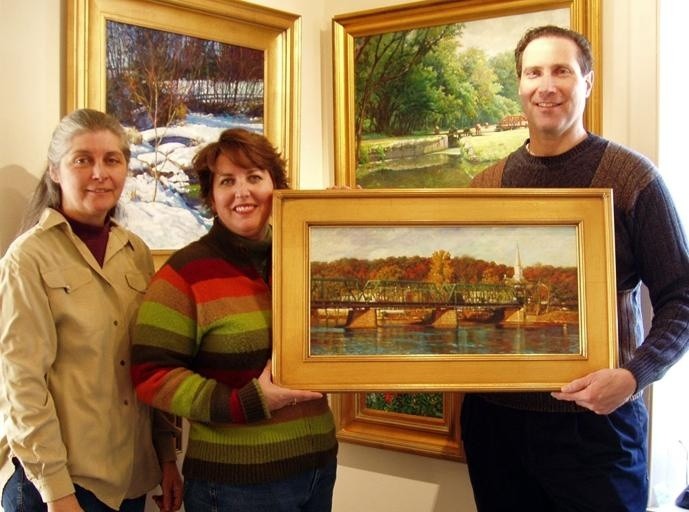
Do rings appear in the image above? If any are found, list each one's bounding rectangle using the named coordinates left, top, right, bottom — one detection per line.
left=292, top=398, right=298, bottom=405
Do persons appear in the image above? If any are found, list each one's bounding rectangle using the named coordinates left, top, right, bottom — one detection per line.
left=128, top=128, right=368, bottom=510
left=0, top=108, right=187, bottom=511
left=450, top=23, right=688, bottom=511
